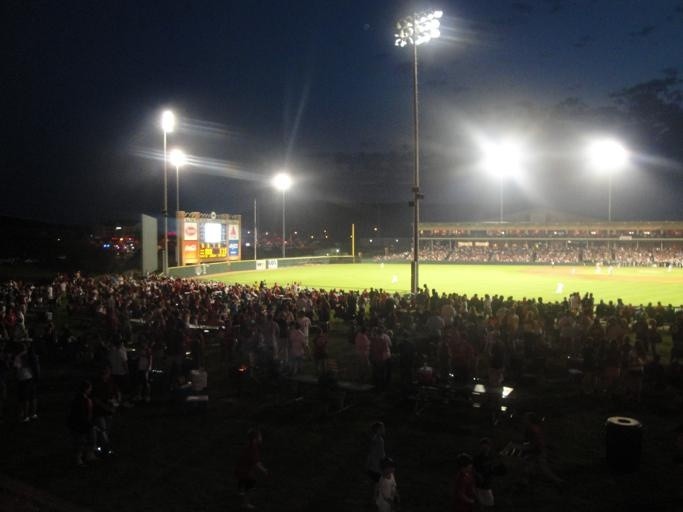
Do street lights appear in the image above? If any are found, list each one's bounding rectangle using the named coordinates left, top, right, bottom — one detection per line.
left=158, top=109, right=176, bottom=275
left=589, top=139, right=626, bottom=223
left=476, top=139, right=519, bottom=223
left=395, top=6, right=448, bottom=298
left=169, top=148, right=188, bottom=265
left=271, top=172, right=294, bottom=257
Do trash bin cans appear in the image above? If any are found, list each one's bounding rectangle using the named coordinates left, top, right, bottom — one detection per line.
left=52, top=254, right=67, bottom=272
left=607, top=416, right=642, bottom=476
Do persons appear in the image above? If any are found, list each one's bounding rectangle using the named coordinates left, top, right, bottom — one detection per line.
left=1, top=270, right=682, bottom=511
left=378, top=241, right=683, bottom=268
